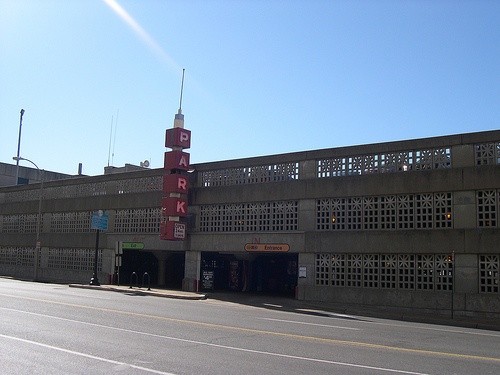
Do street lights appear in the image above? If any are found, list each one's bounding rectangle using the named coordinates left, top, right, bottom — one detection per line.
left=15, top=109, right=25, bottom=185
left=13, top=156, right=43, bottom=282
